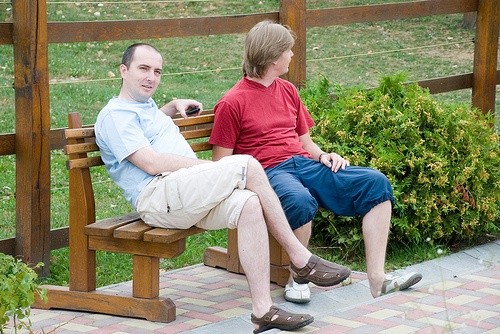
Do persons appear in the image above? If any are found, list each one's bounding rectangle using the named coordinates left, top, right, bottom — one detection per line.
left=94, top=44, right=350, bottom=334
left=208, top=18, right=423, bottom=299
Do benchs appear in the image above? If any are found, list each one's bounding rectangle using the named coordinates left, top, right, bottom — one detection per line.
left=30, top=108, right=292, bottom=323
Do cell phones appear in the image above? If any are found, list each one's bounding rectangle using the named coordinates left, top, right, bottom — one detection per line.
left=186, top=107, right=200, bottom=115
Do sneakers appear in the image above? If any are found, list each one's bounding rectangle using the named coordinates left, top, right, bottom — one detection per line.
left=251, top=305, right=315, bottom=334
left=378, top=272, right=422, bottom=296
left=285, top=281, right=311, bottom=303
left=290, top=254, right=350, bottom=287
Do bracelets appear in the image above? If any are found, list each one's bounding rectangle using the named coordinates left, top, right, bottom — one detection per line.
left=319, top=152, right=326, bottom=162
